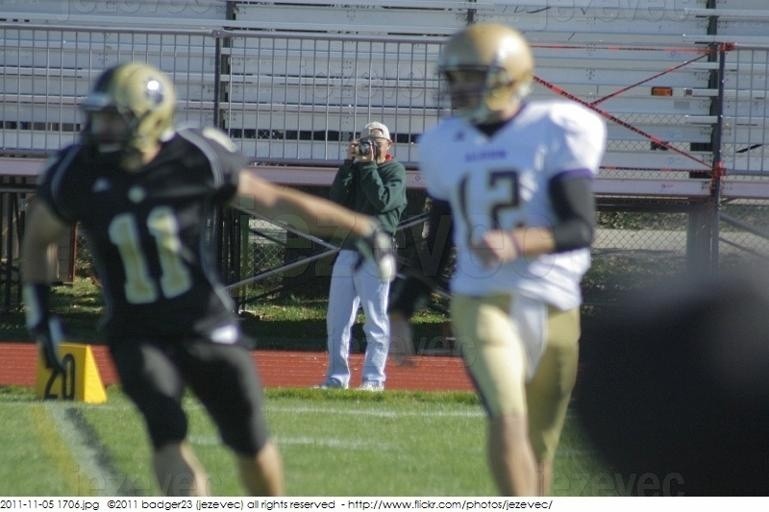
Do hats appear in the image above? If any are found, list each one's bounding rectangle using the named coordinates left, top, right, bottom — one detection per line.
left=360, top=122, right=391, bottom=143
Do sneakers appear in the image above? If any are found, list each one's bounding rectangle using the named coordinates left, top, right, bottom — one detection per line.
left=312, top=378, right=349, bottom=390
left=354, top=381, right=385, bottom=392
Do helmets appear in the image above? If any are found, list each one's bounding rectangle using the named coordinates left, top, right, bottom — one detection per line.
left=433, top=23, right=536, bottom=129
left=74, top=63, right=178, bottom=174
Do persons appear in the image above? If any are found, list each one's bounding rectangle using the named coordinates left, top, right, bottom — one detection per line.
left=19, top=58, right=399, bottom=497
left=311, top=119, right=409, bottom=392
left=387, top=23, right=609, bottom=497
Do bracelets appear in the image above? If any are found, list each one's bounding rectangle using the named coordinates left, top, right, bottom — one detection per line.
left=508, top=229, right=525, bottom=259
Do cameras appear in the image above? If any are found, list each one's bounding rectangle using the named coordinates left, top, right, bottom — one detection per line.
left=359, top=138, right=377, bottom=156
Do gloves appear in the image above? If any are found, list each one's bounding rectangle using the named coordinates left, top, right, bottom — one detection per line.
left=25, top=309, right=75, bottom=376
left=353, top=215, right=400, bottom=285
